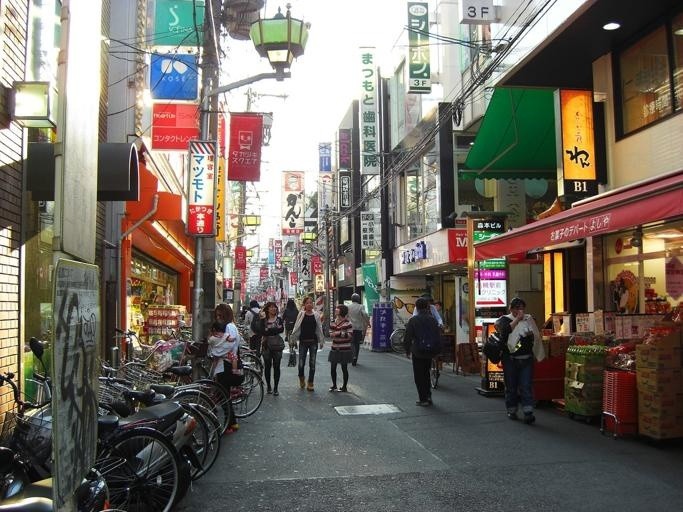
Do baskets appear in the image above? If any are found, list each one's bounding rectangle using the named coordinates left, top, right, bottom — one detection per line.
left=151, top=351, right=173, bottom=372
left=0, top=411, right=51, bottom=466
left=116, top=359, right=162, bottom=392
left=97, top=386, right=120, bottom=416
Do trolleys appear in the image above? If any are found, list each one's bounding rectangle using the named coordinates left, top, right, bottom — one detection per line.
left=599, top=368, right=637, bottom=441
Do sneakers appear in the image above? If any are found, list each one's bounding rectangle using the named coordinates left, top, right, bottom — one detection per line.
left=524, top=415, right=535, bottom=425
left=506, top=413, right=517, bottom=420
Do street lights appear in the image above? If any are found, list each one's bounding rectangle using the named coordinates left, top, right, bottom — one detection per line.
left=231, top=247, right=255, bottom=310
left=225, top=210, right=261, bottom=305
left=279, top=253, right=304, bottom=297
left=299, top=226, right=328, bottom=337
left=192, top=2, right=310, bottom=345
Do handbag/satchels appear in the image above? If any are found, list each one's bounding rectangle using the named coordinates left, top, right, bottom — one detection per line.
left=287, top=346, right=296, bottom=367
left=265, top=335, right=284, bottom=351
left=482, top=332, right=505, bottom=364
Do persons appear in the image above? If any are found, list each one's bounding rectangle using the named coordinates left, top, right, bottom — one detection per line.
left=327, top=304, right=354, bottom=393
left=495, top=298, right=545, bottom=425
left=290, top=297, right=325, bottom=391
left=282, top=299, right=299, bottom=342
left=255, top=301, right=285, bottom=395
left=206, top=300, right=262, bottom=433
left=404, top=294, right=444, bottom=408
left=346, top=293, right=372, bottom=367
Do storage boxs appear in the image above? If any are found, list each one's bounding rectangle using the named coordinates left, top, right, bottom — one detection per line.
left=634, top=334, right=682, bottom=441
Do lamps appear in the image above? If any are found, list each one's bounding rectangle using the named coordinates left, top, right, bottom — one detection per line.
left=5, top=80, right=61, bottom=129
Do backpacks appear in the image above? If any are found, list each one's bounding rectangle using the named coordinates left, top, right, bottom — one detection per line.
left=249, top=309, right=264, bottom=334
left=413, top=314, right=441, bottom=355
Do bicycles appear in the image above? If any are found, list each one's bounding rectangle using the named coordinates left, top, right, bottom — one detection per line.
left=389, top=327, right=407, bottom=355
left=429, top=326, right=447, bottom=390
left=281, top=321, right=296, bottom=353
left=0, top=316, right=265, bottom=512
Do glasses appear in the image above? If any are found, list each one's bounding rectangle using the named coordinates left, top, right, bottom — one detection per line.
left=394, top=296, right=415, bottom=315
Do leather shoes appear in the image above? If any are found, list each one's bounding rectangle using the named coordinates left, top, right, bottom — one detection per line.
left=267, top=387, right=279, bottom=396
left=415, top=401, right=431, bottom=406
left=329, top=385, right=348, bottom=393
left=224, top=423, right=239, bottom=434
left=299, top=376, right=314, bottom=391
left=352, top=357, right=358, bottom=365
